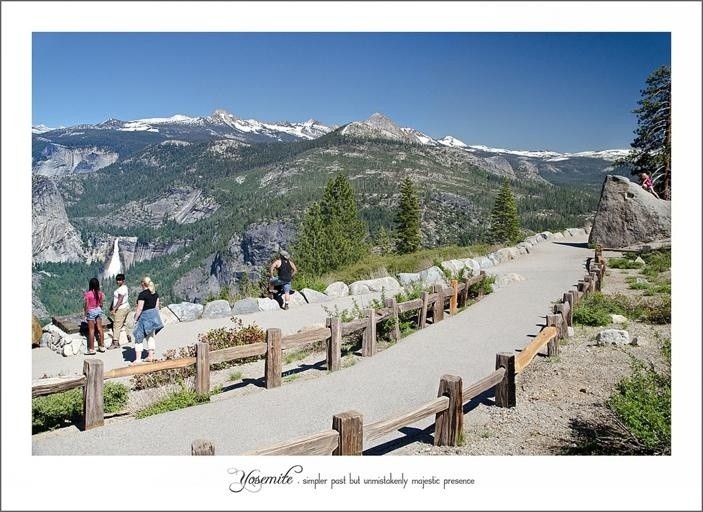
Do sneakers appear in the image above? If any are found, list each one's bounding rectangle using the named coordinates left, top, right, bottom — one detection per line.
left=285, top=304, right=289, bottom=310
left=264, top=291, right=273, bottom=299
left=84, top=340, right=132, bottom=354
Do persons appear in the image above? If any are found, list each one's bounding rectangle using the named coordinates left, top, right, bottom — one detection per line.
left=81, top=278, right=107, bottom=355
left=126, top=276, right=159, bottom=367
left=262, top=250, right=297, bottom=310
left=640, top=173, right=659, bottom=199
left=105, top=273, right=132, bottom=351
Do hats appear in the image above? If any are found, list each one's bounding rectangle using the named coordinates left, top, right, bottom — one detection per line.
left=280, top=250, right=290, bottom=259
